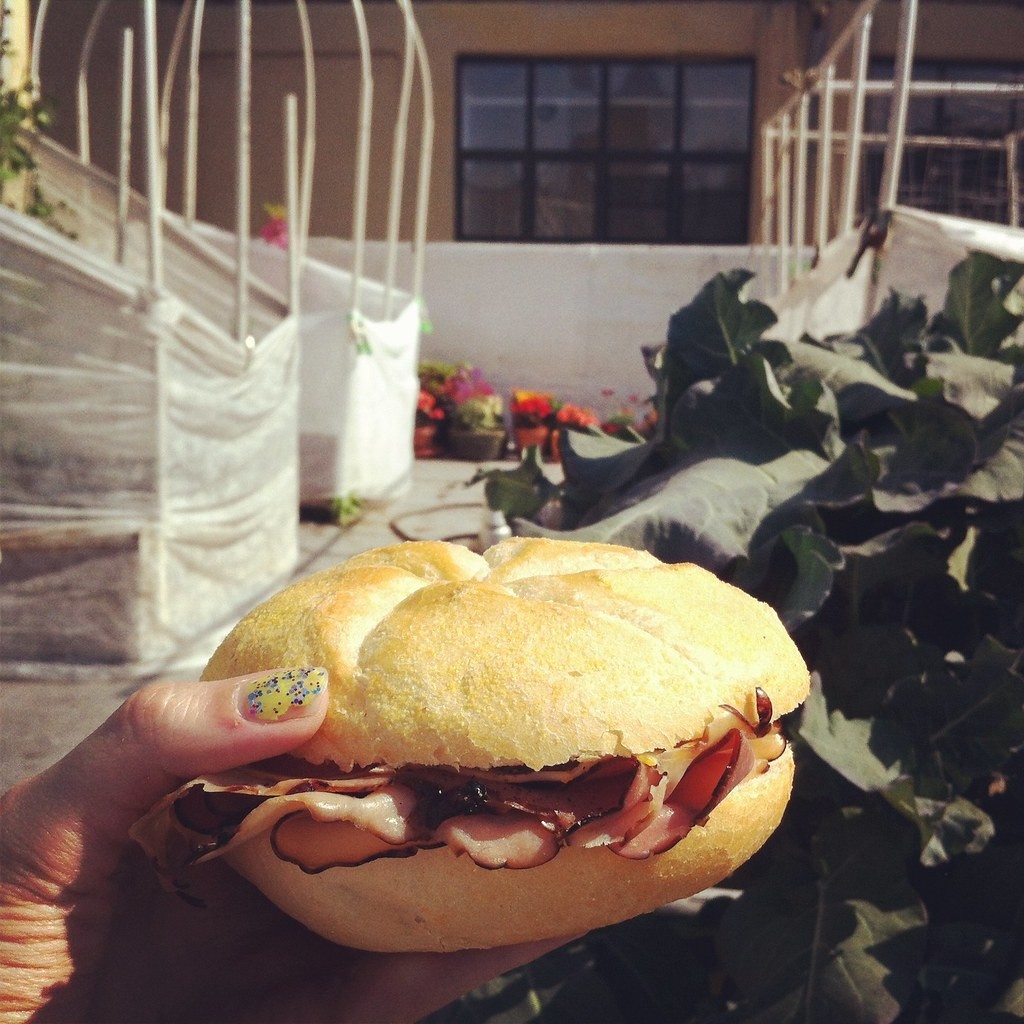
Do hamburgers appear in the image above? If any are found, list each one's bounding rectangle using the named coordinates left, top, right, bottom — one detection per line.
left=130, top=535, right=815, bottom=955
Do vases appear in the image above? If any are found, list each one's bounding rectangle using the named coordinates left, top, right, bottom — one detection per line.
left=443, top=429, right=507, bottom=461
left=513, top=425, right=549, bottom=456
left=413, top=422, right=447, bottom=458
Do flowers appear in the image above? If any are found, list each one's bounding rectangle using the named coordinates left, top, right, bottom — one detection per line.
left=421, top=359, right=663, bottom=428
